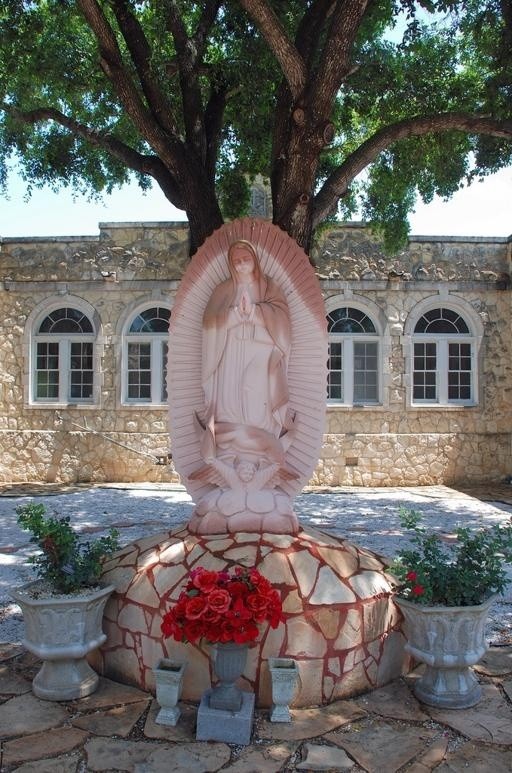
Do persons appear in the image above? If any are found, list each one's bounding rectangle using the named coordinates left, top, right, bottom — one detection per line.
left=192, top=238, right=296, bottom=454
left=204, top=456, right=280, bottom=492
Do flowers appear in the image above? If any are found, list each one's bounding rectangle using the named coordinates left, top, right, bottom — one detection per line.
left=161, top=565, right=286, bottom=647
left=391, top=504, right=511, bottom=602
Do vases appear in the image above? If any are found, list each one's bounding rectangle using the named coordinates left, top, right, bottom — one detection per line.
left=208, top=643, right=248, bottom=711
left=395, top=599, right=489, bottom=710
left=153, top=660, right=187, bottom=725
left=269, top=659, right=298, bottom=723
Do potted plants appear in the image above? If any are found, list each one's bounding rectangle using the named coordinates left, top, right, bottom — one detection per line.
left=15, top=503, right=120, bottom=699
